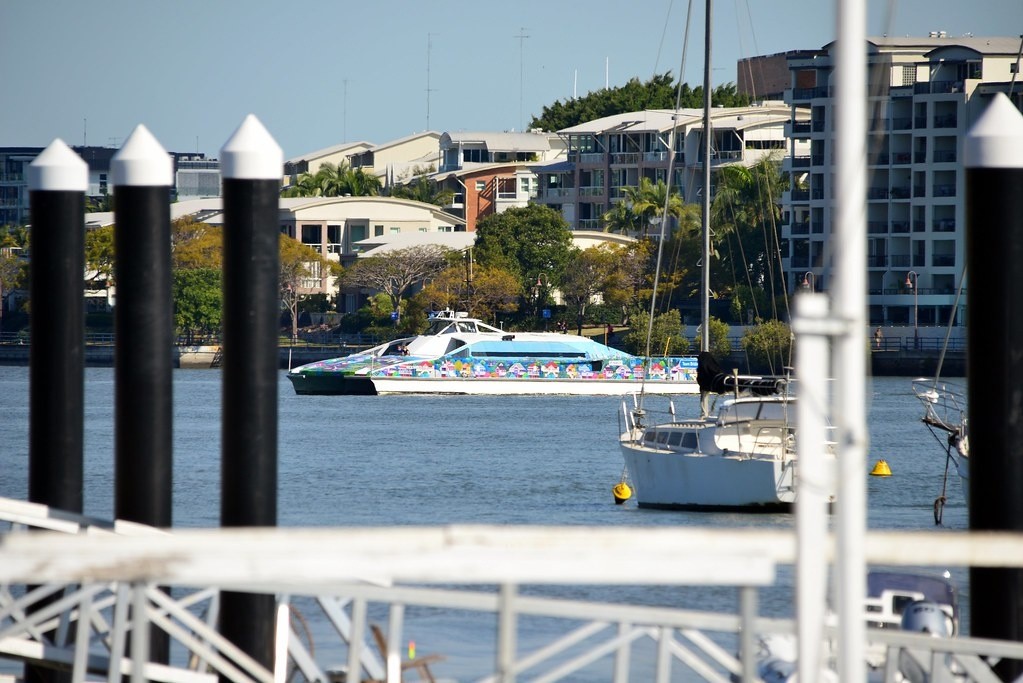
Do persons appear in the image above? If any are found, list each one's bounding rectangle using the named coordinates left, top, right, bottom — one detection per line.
left=607, top=322, right=613, bottom=338
left=874, top=328, right=882, bottom=347
left=396, top=346, right=411, bottom=356
left=564, top=327, right=568, bottom=335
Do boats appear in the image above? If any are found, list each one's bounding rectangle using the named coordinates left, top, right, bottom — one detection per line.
left=287, top=316, right=701, bottom=395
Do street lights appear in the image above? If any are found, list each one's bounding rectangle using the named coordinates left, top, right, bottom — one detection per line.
left=462, top=249, right=469, bottom=313
left=537, top=273, right=548, bottom=332
left=802, top=271, right=814, bottom=292
left=905, top=271, right=919, bottom=351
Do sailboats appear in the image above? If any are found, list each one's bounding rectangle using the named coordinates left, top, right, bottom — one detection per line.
left=618, top=0, right=795, bottom=514
left=910, top=34, right=1023, bottom=513
